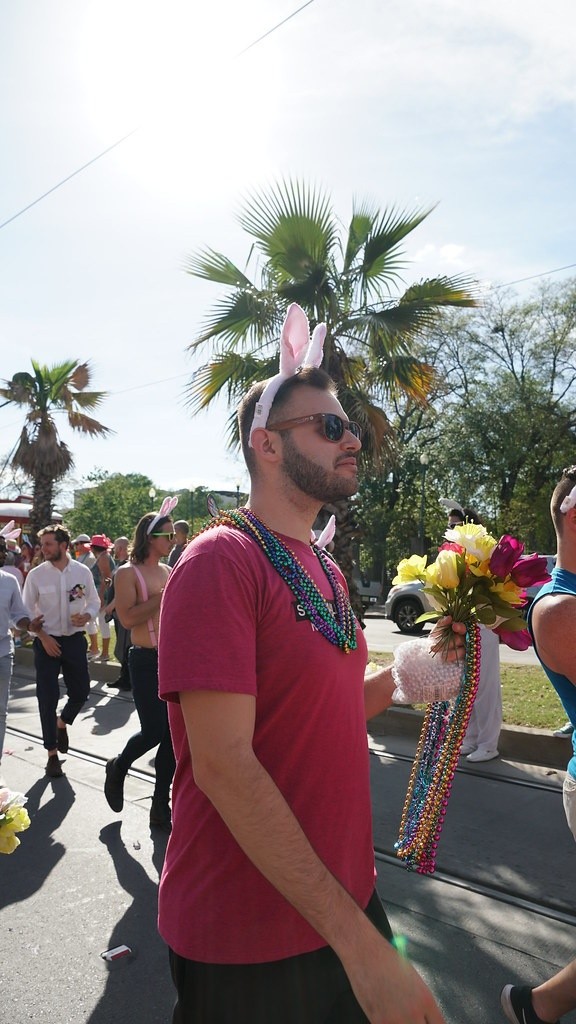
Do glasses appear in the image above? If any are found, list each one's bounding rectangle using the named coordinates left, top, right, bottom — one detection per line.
left=152, top=532, right=177, bottom=541
left=447, top=521, right=462, bottom=528
left=270, top=411, right=361, bottom=442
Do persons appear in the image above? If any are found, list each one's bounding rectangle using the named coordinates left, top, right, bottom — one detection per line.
left=6, top=540, right=44, bottom=649
left=22, top=526, right=102, bottom=777
left=499, top=476, right=576, bottom=1024
left=442, top=499, right=503, bottom=761
left=0, top=535, right=44, bottom=762
left=553, top=722, right=573, bottom=739
left=103, top=510, right=179, bottom=835
left=168, top=520, right=191, bottom=566
left=68, top=533, right=132, bottom=692
left=155, top=301, right=469, bottom=1024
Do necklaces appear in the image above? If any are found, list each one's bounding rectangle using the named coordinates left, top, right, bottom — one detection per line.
left=393, top=620, right=482, bottom=877
left=190, top=504, right=358, bottom=656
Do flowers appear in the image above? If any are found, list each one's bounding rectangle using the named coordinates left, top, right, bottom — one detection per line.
left=0, top=784, right=31, bottom=854
left=392, top=515, right=553, bottom=667
left=67, top=583, right=86, bottom=603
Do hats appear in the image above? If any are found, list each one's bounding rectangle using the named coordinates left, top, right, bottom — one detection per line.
left=4, top=539, right=22, bottom=554
left=70, top=533, right=90, bottom=544
left=84, top=533, right=115, bottom=548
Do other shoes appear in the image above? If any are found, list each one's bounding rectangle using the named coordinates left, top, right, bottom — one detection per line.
left=461, top=745, right=474, bottom=755
left=86, top=650, right=101, bottom=659
left=55, top=721, right=68, bottom=754
left=46, top=760, right=63, bottom=778
left=150, top=808, right=171, bottom=824
left=466, top=746, right=498, bottom=762
left=554, top=721, right=574, bottom=738
left=105, top=757, right=128, bottom=813
left=107, top=677, right=131, bottom=692
left=91, top=653, right=109, bottom=661
left=15, top=641, right=21, bottom=647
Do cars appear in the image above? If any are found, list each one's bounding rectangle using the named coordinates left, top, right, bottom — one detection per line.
left=383, top=555, right=556, bottom=634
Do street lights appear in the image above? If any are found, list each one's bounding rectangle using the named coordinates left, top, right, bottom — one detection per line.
left=419, top=450, right=430, bottom=558
left=234, top=478, right=242, bottom=510
left=148, top=487, right=157, bottom=512
left=188, top=482, right=196, bottom=536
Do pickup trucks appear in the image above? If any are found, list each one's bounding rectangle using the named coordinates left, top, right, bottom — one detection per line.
left=354, top=580, right=382, bottom=615
